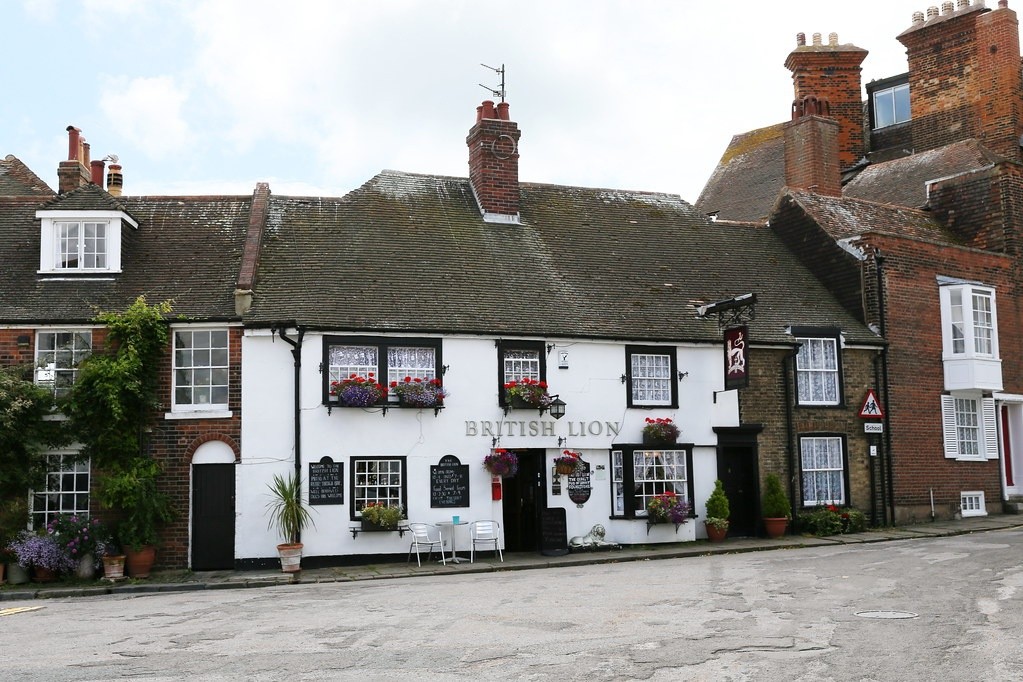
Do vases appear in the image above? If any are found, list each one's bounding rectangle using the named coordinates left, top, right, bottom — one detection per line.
left=807, top=522, right=817, bottom=535
left=648, top=510, right=668, bottom=523
left=338, top=395, right=386, bottom=406
left=361, top=518, right=398, bottom=530
left=643, top=432, right=676, bottom=446
left=76, top=553, right=95, bottom=579
left=33, top=566, right=56, bottom=581
left=7, top=563, right=28, bottom=584
left=505, top=398, right=540, bottom=408
left=556, top=465, right=573, bottom=474
left=101, top=555, right=126, bottom=577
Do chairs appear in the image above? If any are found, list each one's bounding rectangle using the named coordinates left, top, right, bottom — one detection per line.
left=469, top=520, right=503, bottom=563
left=407, top=523, right=445, bottom=567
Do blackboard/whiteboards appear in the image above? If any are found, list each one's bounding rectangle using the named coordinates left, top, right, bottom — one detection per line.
left=540, top=508, right=568, bottom=549
left=431, top=455, right=470, bottom=508
left=309, top=456, right=343, bottom=505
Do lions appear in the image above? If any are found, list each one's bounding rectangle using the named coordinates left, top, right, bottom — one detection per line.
left=570, top=524, right=618, bottom=548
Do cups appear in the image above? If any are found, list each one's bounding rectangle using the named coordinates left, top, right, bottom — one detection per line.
left=452, top=515, right=459, bottom=524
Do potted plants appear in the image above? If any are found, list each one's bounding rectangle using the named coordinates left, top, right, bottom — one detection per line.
left=761, top=472, right=788, bottom=537
left=93, top=459, right=180, bottom=579
left=704, top=480, right=730, bottom=543
left=841, top=507, right=871, bottom=534
left=264, top=472, right=318, bottom=572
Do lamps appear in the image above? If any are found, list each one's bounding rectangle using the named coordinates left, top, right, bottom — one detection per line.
left=539, top=395, right=566, bottom=419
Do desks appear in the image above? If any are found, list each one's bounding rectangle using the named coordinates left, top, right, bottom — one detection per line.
left=436, top=521, right=470, bottom=564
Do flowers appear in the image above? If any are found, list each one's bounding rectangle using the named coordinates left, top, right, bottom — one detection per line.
left=642, top=418, right=680, bottom=440
left=798, top=503, right=843, bottom=537
left=391, top=376, right=445, bottom=406
left=504, top=378, right=548, bottom=406
left=554, top=450, right=578, bottom=467
left=2, top=511, right=117, bottom=576
left=646, top=491, right=691, bottom=524
left=483, top=449, right=519, bottom=478
left=361, top=500, right=405, bottom=530
left=331, top=372, right=389, bottom=405
